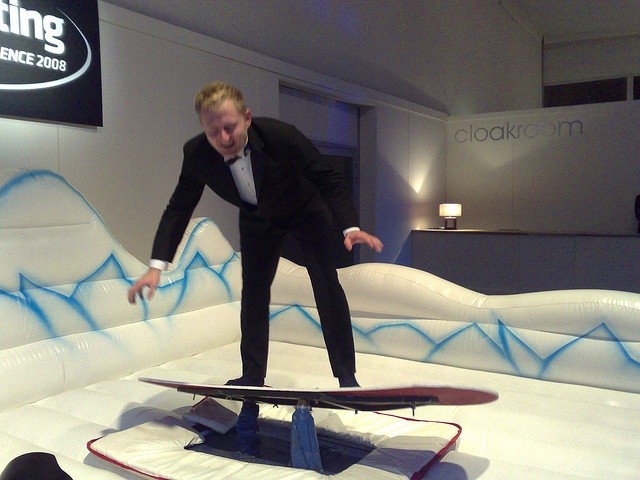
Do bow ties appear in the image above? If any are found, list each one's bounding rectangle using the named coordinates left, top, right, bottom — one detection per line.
left=221, top=145, right=250, bottom=169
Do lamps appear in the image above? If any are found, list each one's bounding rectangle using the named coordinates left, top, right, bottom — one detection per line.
left=439, top=203, right=462, bottom=230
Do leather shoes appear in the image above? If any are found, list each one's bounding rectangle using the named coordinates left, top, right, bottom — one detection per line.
left=225, top=376, right=264, bottom=388
left=338, top=376, right=364, bottom=389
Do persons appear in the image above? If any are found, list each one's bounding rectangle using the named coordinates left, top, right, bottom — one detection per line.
left=129, top=83, right=384, bottom=388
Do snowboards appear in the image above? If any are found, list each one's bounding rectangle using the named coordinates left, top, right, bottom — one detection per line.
left=138, top=378, right=500, bottom=411
